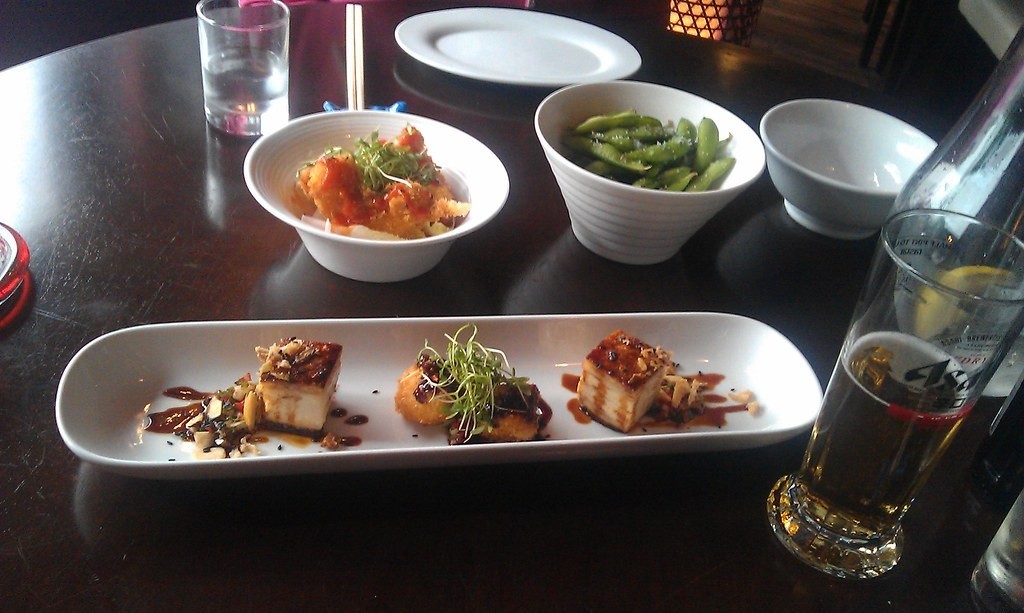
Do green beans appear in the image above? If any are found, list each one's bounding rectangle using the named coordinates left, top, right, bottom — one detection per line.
left=560, top=109, right=736, bottom=193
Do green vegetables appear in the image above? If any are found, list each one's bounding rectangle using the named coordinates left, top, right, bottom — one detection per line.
left=418, top=321, right=530, bottom=442
left=299, top=129, right=437, bottom=189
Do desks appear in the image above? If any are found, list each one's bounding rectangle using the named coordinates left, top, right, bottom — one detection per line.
left=0, top=0, right=1024, bottom=613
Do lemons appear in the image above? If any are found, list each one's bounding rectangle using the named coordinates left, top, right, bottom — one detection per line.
left=914, top=266, right=1024, bottom=338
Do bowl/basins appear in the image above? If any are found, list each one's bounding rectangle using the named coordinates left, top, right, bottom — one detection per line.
left=534, top=79, right=768, bottom=265
left=759, top=98, right=939, bottom=241
left=243, top=108, right=511, bottom=282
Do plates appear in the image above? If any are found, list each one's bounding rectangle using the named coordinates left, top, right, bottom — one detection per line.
left=394, top=7, right=643, bottom=87
left=55, top=311, right=825, bottom=480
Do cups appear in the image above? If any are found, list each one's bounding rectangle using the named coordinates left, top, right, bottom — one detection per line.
left=970, top=485, right=1024, bottom=613
left=767, top=207, right=1024, bottom=579
left=196, top=0, right=290, bottom=136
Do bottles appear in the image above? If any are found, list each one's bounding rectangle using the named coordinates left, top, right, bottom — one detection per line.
left=850, top=23, right=1024, bottom=382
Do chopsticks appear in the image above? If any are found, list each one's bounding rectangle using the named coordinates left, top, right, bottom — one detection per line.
left=346, top=3, right=364, bottom=111
